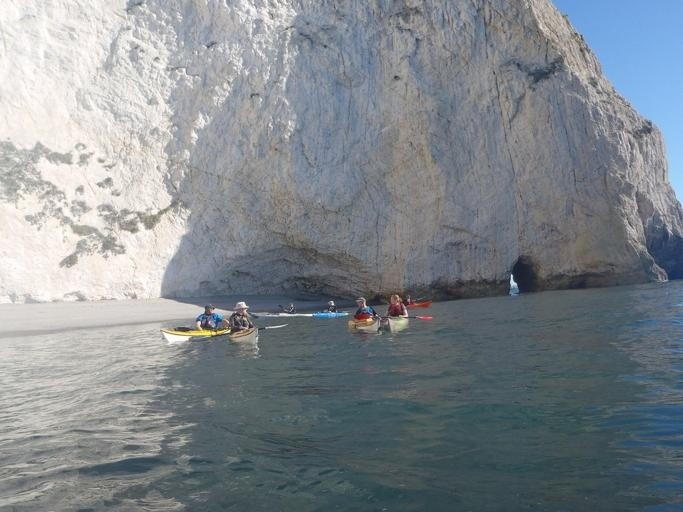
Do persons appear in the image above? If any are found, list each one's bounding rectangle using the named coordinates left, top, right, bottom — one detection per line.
left=324, top=301, right=336, bottom=312
left=195, top=304, right=231, bottom=331
left=388, top=294, right=408, bottom=318
left=354, top=297, right=378, bottom=318
left=229, top=302, right=254, bottom=334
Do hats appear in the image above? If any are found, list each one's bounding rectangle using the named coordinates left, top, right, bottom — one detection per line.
left=232, top=301, right=250, bottom=310
left=205, top=304, right=215, bottom=313
left=328, top=301, right=334, bottom=305
left=355, top=297, right=366, bottom=302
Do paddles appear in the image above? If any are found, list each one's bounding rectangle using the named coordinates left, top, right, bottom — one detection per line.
left=174, top=327, right=197, bottom=332
left=353, top=314, right=433, bottom=320
left=257, top=324, right=290, bottom=331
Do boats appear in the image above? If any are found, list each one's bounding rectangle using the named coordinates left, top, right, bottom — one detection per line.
left=160, top=299, right=434, bottom=342
